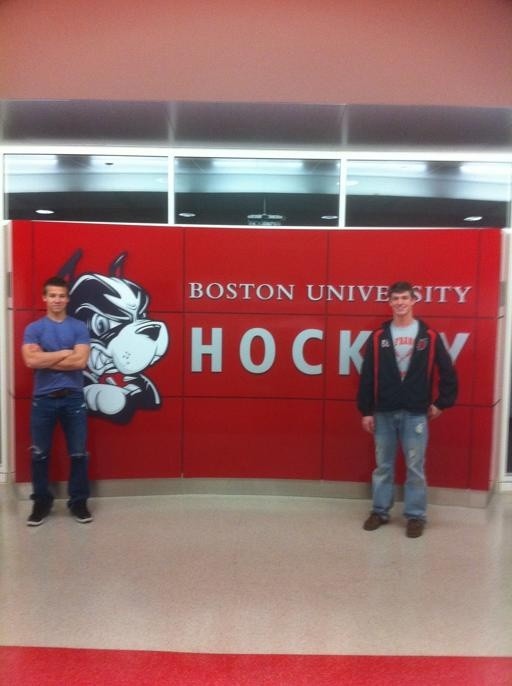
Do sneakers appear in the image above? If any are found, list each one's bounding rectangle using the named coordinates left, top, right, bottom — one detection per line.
left=406, top=519, right=425, bottom=538
left=26, top=504, right=55, bottom=526
left=364, top=513, right=388, bottom=531
left=64, top=499, right=94, bottom=523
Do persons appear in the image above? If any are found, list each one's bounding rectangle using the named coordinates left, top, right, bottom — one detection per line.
left=355, top=281, right=459, bottom=538
left=22, top=276, right=94, bottom=527
